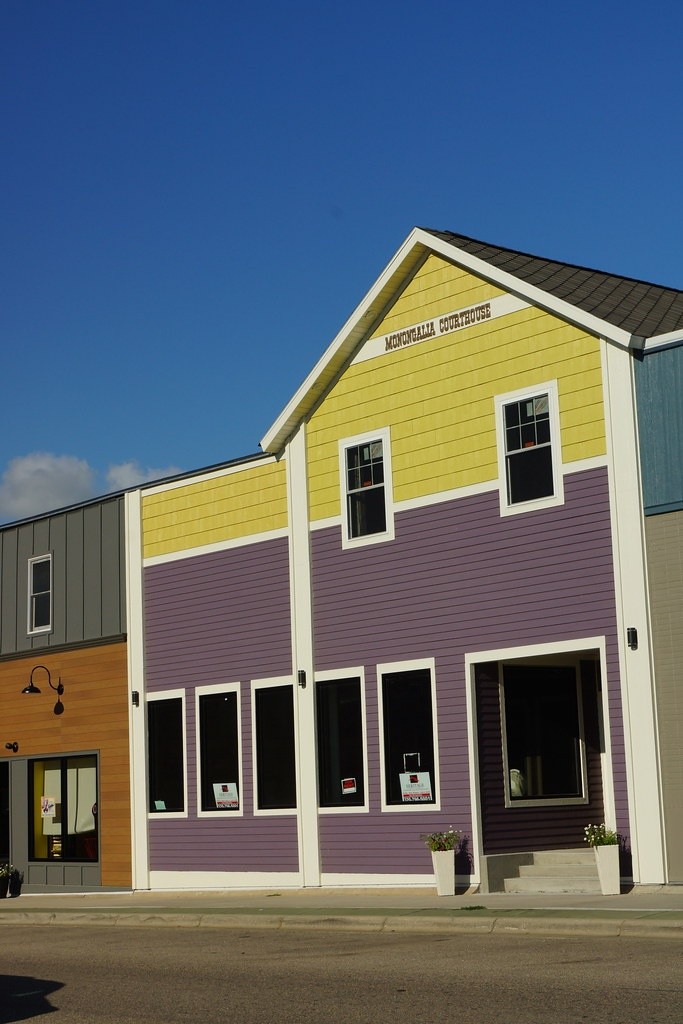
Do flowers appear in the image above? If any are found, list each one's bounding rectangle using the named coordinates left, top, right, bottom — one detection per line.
left=419, top=826, right=462, bottom=852
left=583, top=823, right=621, bottom=847
left=0, top=863, right=13, bottom=877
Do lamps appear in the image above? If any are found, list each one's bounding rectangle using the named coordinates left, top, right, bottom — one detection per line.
left=22, top=666, right=64, bottom=695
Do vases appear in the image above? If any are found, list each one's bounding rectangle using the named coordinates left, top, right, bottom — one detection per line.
left=0, top=877, right=9, bottom=898
left=593, top=844, right=620, bottom=896
left=430, top=850, right=455, bottom=896
